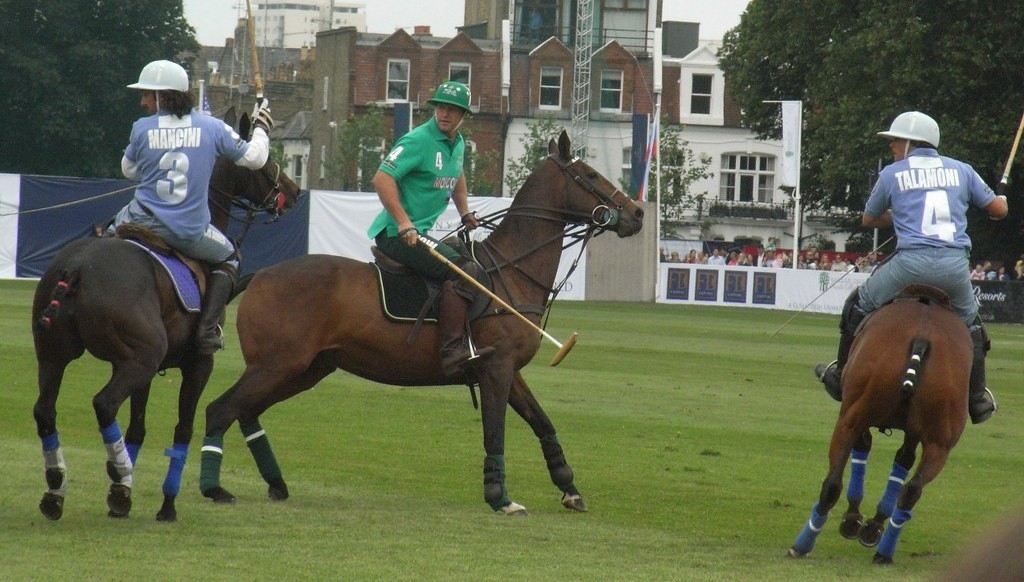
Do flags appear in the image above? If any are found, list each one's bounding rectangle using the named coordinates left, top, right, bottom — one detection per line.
left=637, top=111, right=657, bottom=202
left=203, top=88, right=211, bottom=115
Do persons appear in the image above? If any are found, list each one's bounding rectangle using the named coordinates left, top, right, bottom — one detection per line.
left=968, top=258, right=1024, bottom=282
left=101, top=60, right=273, bottom=355
left=366, top=81, right=496, bottom=378
left=659, top=236, right=877, bottom=273
left=815, top=110, right=1008, bottom=424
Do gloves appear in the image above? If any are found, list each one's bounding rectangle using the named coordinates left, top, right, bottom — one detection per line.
left=251, top=99, right=274, bottom=134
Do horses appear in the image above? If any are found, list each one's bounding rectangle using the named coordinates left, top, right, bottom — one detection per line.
left=783, top=284, right=981, bottom=568
left=30, top=104, right=303, bottom=522
left=199, top=129, right=645, bottom=518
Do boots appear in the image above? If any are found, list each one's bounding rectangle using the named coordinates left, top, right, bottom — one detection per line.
left=193, top=272, right=233, bottom=354
left=440, top=281, right=496, bottom=380
left=968, top=328, right=996, bottom=424
left=815, top=309, right=865, bottom=403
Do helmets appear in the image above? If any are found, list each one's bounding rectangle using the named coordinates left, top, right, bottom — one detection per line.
left=426, top=81, right=472, bottom=113
left=877, top=111, right=940, bottom=147
left=126, top=59, right=189, bottom=92
